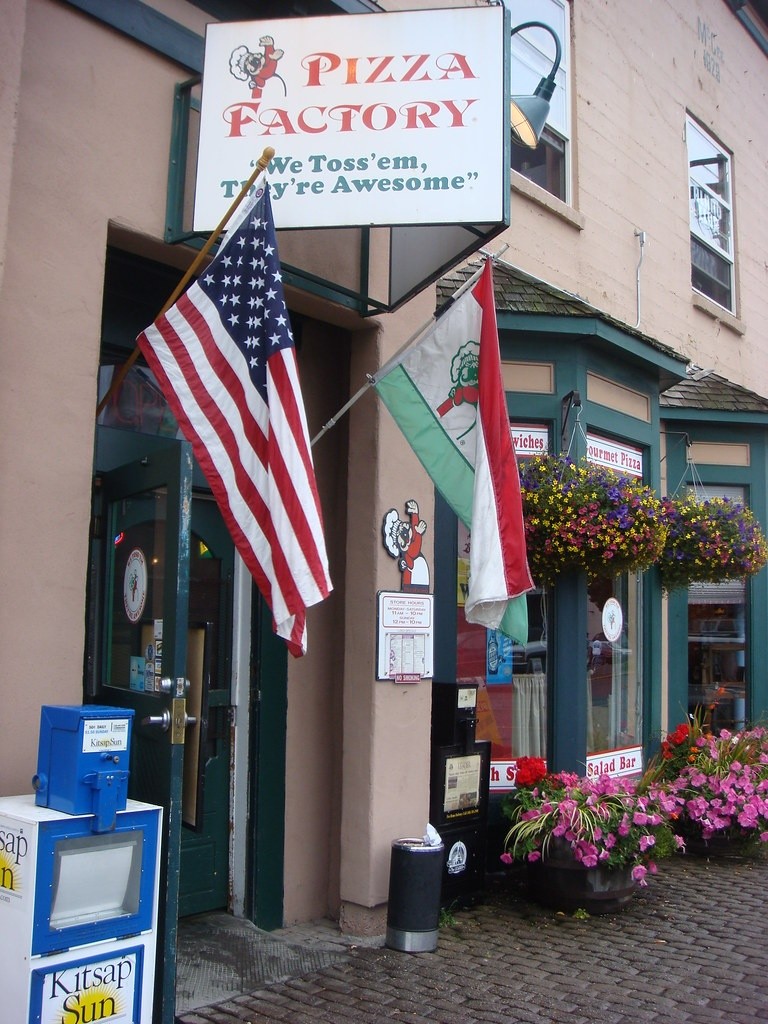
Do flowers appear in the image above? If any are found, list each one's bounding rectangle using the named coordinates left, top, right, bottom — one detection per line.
left=501, top=754, right=687, bottom=889
left=658, top=685, right=768, bottom=845
left=518, top=451, right=670, bottom=584
left=659, top=484, right=768, bottom=586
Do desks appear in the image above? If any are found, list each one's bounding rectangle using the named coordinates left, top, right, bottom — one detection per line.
left=512, top=671, right=594, bottom=758
left=688, top=635, right=745, bottom=729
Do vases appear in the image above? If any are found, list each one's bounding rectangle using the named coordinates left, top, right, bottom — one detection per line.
left=677, top=835, right=728, bottom=858
left=527, top=842, right=637, bottom=914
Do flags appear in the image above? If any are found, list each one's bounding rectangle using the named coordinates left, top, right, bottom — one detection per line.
left=373, top=260, right=535, bottom=645
left=138, top=182, right=335, bottom=658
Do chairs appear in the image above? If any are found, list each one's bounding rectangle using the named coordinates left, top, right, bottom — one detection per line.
left=705, top=643, right=745, bottom=733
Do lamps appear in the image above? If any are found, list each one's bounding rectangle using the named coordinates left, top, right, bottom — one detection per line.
left=510, top=21, right=563, bottom=149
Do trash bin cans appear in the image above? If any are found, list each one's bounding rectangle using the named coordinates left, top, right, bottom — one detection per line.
left=385, top=837, right=447, bottom=953
